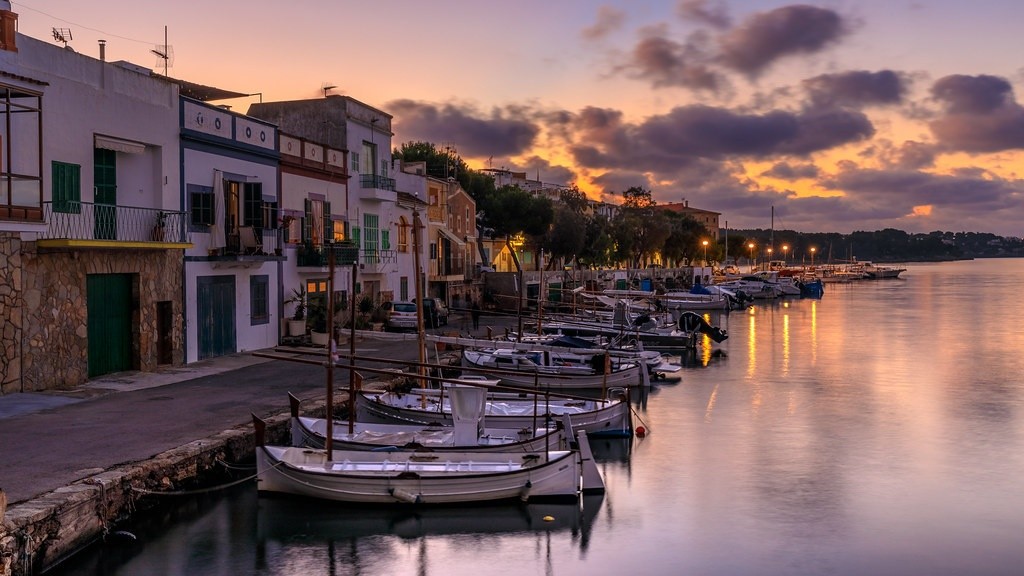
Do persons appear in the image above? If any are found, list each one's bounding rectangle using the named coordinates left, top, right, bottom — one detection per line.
left=471, top=301, right=480, bottom=330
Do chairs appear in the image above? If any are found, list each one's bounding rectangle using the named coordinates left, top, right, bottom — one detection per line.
left=237, top=226, right=264, bottom=255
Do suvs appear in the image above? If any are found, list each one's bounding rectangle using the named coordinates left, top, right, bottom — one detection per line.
left=414, top=296, right=449, bottom=328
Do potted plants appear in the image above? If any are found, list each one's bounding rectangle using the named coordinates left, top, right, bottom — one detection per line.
left=358, top=295, right=374, bottom=323
left=274, top=215, right=296, bottom=254
left=149, top=211, right=168, bottom=241
left=284, top=281, right=342, bottom=344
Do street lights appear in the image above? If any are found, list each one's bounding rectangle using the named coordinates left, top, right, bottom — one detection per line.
left=702, top=240, right=709, bottom=266
left=783, top=245, right=788, bottom=268
left=768, top=249, right=771, bottom=262
left=749, top=243, right=754, bottom=274
left=811, top=247, right=816, bottom=267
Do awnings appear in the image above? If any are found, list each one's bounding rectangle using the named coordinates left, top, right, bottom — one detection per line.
left=437, top=227, right=466, bottom=250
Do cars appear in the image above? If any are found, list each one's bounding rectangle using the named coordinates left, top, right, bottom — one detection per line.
left=380, top=300, right=419, bottom=332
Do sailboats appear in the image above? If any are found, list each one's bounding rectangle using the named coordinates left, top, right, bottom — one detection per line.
left=252, top=205, right=908, bottom=506
